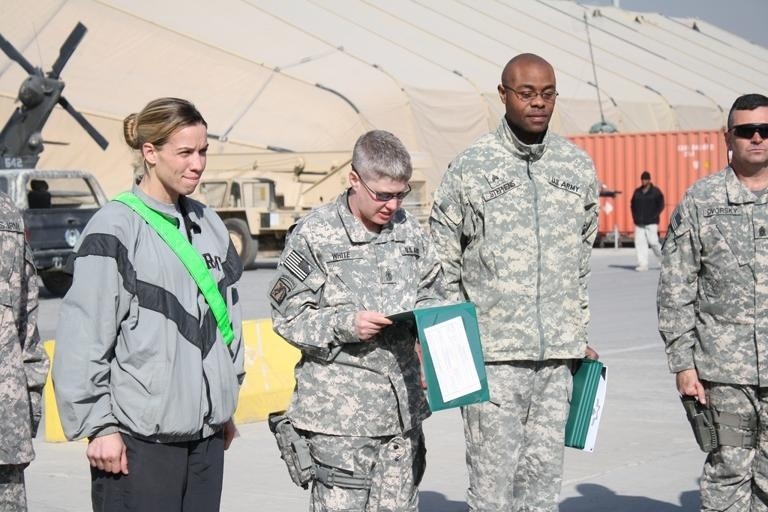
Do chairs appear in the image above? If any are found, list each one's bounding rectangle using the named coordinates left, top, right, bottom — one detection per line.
left=27, top=180, right=52, bottom=208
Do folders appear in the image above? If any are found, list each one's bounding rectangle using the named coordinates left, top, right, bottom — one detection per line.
left=372, top=304, right=491, bottom=412
left=565, top=358, right=604, bottom=450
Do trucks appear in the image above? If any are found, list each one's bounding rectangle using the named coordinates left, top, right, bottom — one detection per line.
left=561, top=131, right=728, bottom=244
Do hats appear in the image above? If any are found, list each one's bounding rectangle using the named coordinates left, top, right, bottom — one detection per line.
left=641, top=172, right=650, bottom=180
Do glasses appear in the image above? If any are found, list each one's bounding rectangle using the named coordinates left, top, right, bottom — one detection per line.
left=503, top=85, right=558, bottom=102
left=352, top=163, right=411, bottom=200
left=728, top=123, right=768, bottom=139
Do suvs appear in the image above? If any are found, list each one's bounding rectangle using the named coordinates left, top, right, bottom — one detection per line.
left=1, top=168, right=106, bottom=295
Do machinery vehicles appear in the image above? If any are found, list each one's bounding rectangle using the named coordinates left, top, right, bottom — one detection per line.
left=183, top=152, right=432, bottom=268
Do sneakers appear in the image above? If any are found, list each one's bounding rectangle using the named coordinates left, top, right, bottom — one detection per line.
left=636, top=267, right=648, bottom=271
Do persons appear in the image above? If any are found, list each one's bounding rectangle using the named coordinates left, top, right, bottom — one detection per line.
left=658, top=93, right=768, bottom=511
left=431, top=52, right=598, bottom=512
left=268, top=129, right=442, bottom=512
left=0, top=188, right=53, bottom=512
left=630, top=170, right=662, bottom=270
left=52, top=96, right=245, bottom=512
